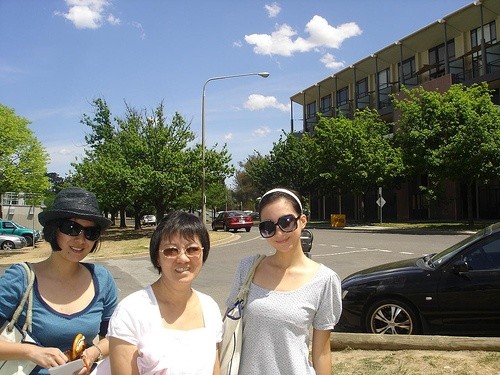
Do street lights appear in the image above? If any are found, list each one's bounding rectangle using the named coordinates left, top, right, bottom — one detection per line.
left=202, top=71, right=270, bottom=227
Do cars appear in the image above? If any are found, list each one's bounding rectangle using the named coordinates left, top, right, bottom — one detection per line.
left=331, top=221, right=500, bottom=337
left=0, top=220, right=40, bottom=250
left=212, top=210, right=259, bottom=232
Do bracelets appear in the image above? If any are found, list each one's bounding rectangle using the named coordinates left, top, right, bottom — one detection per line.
left=93, top=344, right=103, bottom=360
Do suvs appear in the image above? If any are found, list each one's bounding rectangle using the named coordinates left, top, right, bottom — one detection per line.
left=140, top=215, right=157, bottom=226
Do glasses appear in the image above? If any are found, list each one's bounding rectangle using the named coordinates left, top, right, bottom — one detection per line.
left=158, top=246, right=204, bottom=258
left=258, top=214, right=302, bottom=238
left=55, top=220, right=101, bottom=241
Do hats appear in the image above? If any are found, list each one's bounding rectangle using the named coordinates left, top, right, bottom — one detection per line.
left=38, top=187, right=113, bottom=234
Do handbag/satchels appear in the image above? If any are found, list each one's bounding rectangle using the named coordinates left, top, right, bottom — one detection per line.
left=218, top=254, right=265, bottom=375
left=0, top=262, right=40, bottom=375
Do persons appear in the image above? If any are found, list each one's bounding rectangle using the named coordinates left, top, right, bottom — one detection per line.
left=107, top=211, right=223, bottom=375
left=225, top=188, right=342, bottom=375
left=0, top=187, right=118, bottom=375
